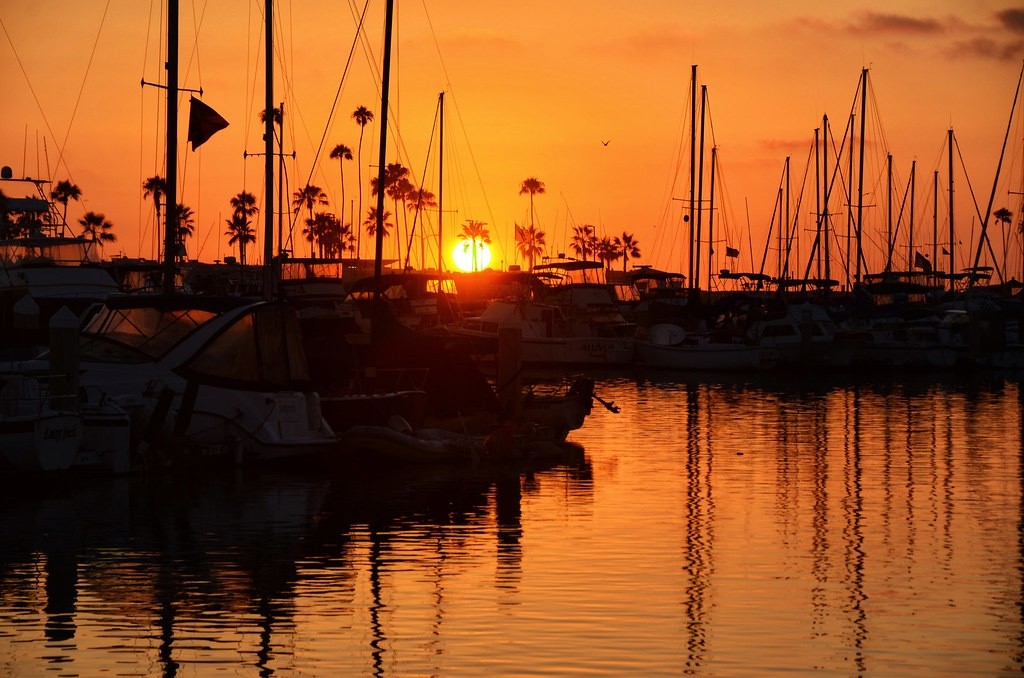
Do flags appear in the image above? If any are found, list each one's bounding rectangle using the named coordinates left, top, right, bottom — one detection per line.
left=188, top=96, right=228, bottom=153
left=914, top=251, right=932, bottom=268
left=726, top=246, right=739, bottom=259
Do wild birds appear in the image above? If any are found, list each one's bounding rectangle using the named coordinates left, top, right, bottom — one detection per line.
left=601, top=140, right=610, bottom=146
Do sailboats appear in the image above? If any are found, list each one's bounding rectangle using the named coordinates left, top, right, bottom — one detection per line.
left=642, top=65, right=1024, bottom=372
left=0, top=0, right=594, bottom=480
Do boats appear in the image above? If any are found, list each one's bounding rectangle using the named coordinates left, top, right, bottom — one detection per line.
left=444, top=285, right=638, bottom=371
